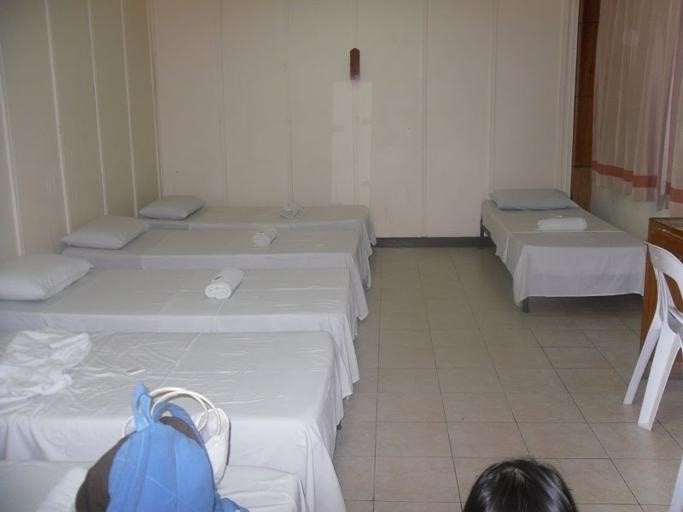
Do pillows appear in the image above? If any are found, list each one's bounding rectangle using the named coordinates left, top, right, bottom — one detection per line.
left=139, top=194, right=205, bottom=221
left=489, top=189, right=579, bottom=210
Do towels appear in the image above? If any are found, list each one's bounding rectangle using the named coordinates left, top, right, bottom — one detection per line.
left=537, top=217, right=587, bottom=232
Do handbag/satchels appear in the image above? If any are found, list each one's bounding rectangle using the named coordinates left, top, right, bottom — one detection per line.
left=122, top=408, right=230, bottom=484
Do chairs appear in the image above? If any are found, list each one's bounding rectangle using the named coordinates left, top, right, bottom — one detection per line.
left=621, top=241, right=682, bottom=431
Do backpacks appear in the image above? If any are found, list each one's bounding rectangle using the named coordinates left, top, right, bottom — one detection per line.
left=105, top=383, right=246, bottom=512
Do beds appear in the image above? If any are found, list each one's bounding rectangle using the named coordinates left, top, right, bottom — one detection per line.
left=478, top=197, right=648, bottom=313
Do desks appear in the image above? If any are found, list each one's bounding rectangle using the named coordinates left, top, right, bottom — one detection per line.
left=638, top=216, right=683, bottom=362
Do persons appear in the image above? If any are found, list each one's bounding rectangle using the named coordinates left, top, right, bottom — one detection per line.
left=461, top=457, right=582, bottom=512
